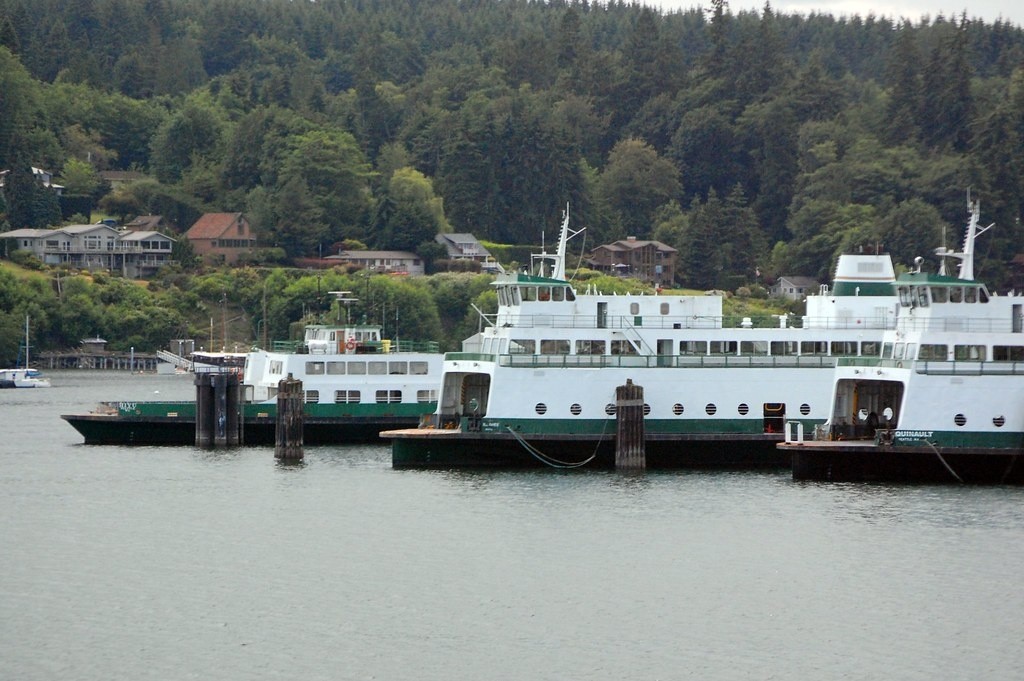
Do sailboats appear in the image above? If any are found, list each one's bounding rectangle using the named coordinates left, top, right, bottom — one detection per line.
left=0, top=316, right=51, bottom=388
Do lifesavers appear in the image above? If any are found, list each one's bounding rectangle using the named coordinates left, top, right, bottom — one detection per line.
left=345, top=341, right=355, bottom=350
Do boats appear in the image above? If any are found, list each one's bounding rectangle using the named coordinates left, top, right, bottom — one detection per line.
left=378, top=196, right=1022, bottom=471
left=61, top=324, right=440, bottom=447
